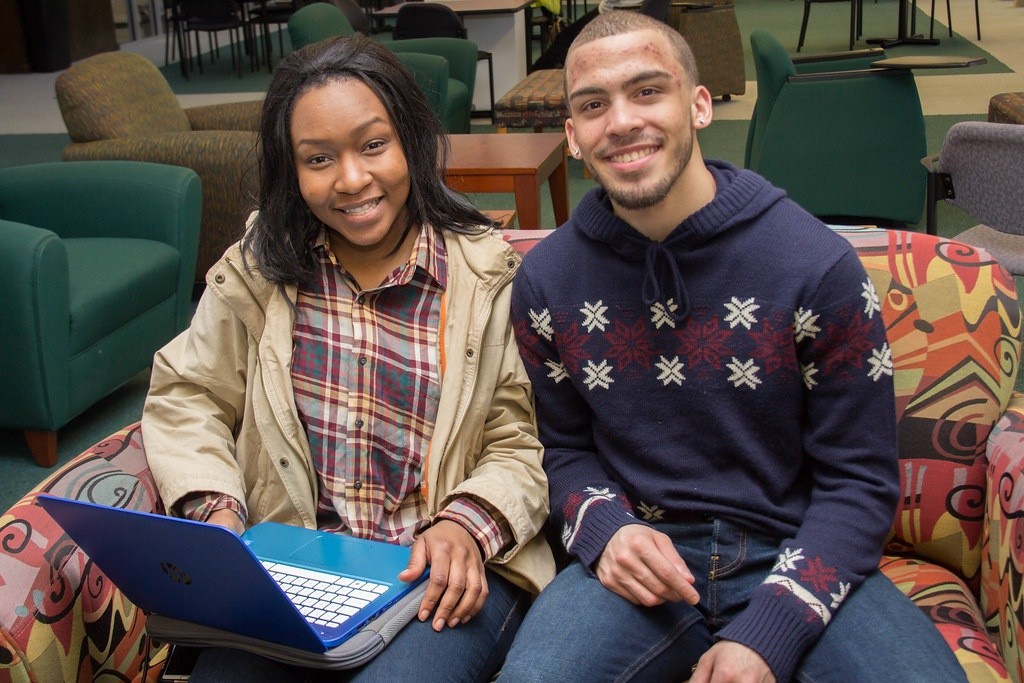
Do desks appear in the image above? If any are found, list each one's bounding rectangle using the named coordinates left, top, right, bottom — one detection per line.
left=372, top=0, right=539, bottom=29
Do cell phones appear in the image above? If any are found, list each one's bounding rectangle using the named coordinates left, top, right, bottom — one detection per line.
left=161, top=645, right=204, bottom=681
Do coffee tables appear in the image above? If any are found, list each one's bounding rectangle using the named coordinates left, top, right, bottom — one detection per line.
left=432, top=131, right=570, bottom=231
left=494, top=68, right=594, bottom=180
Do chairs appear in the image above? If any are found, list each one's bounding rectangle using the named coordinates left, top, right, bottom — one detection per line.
left=921, top=121, right=1024, bottom=275
left=162, top=0, right=372, bottom=72
left=392, top=2, right=496, bottom=125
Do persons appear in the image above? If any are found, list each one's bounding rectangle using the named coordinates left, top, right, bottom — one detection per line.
left=141, top=34, right=557, bottom=683
left=511, top=7, right=973, bottom=683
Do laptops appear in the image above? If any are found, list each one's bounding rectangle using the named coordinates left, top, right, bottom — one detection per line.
left=37, top=493, right=432, bottom=655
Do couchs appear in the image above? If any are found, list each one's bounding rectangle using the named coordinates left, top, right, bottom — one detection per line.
left=744, top=30, right=930, bottom=230
left=54, top=50, right=268, bottom=294
left=289, top=3, right=478, bottom=134
left=614, top=1, right=746, bottom=102
left=1, top=159, right=201, bottom=467
left=0, top=226, right=1024, bottom=683
